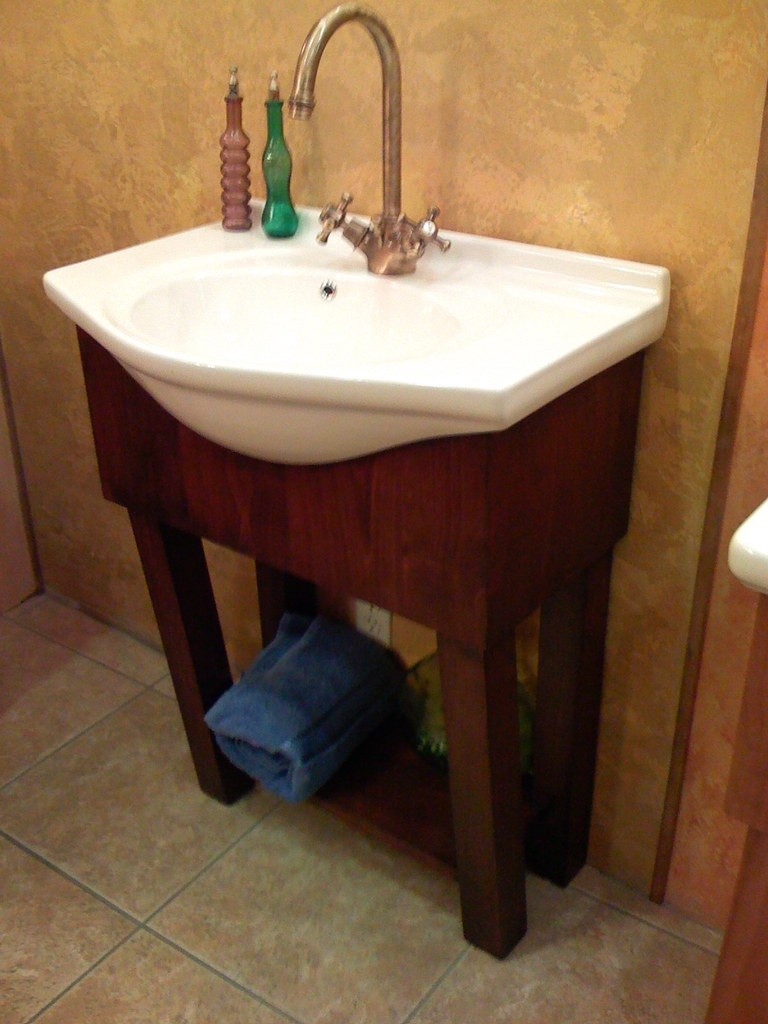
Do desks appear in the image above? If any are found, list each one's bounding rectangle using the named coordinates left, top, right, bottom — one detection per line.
left=75, top=326, right=645, bottom=961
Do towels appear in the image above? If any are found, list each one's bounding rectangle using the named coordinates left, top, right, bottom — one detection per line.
left=202, top=611, right=407, bottom=805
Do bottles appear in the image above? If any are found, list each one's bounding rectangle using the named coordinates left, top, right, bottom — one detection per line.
left=261, top=71, right=299, bottom=239
left=219, top=68, right=253, bottom=231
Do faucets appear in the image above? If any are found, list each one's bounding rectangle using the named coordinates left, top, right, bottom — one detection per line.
left=285, top=4, right=452, bottom=275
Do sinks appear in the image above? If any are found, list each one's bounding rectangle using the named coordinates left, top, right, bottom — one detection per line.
left=42, top=192, right=671, bottom=465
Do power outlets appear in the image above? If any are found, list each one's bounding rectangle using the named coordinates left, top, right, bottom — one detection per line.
left=355, top=598, right=392, bottom=649
